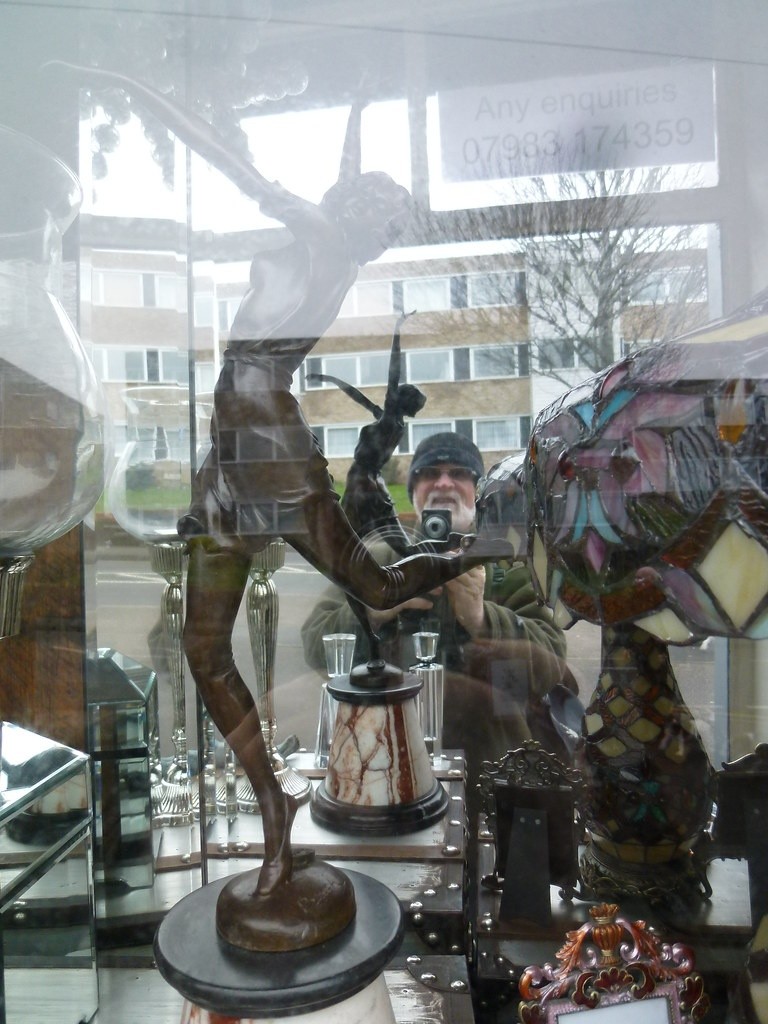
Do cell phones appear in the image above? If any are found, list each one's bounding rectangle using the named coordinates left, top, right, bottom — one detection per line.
left=420, top=509, right=451, bottom=544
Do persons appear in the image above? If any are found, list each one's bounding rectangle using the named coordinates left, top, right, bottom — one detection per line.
left=43, top=53, right=516, bottom=899
left=305, top=310, right=470, bottom=667
left=300, top=432, right=567, bottom=762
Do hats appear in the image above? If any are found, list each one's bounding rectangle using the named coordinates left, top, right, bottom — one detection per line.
left=408, top=432, right=484, bottom=503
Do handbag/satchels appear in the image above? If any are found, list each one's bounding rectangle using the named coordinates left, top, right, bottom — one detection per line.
left=529, top=664, right=588, bottom=768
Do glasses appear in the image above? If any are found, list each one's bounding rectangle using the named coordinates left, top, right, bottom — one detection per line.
left=415, top=466, right=478, bottom=483
